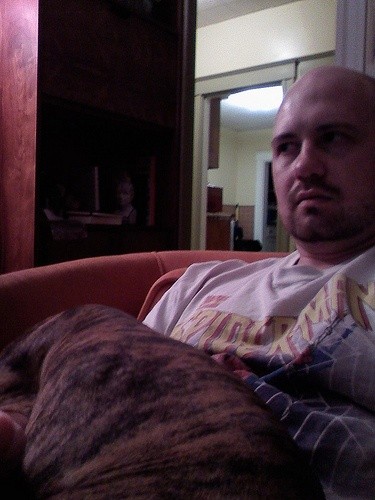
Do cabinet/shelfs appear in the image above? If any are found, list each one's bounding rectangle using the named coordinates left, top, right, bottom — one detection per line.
left=0, top=0, right=196, bottom=274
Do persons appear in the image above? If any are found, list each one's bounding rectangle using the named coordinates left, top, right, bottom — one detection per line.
left=0, top=65, right=375, bottom=500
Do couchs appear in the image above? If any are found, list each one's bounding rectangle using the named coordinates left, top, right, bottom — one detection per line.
left=0, top=250, right=290, bottom=351
